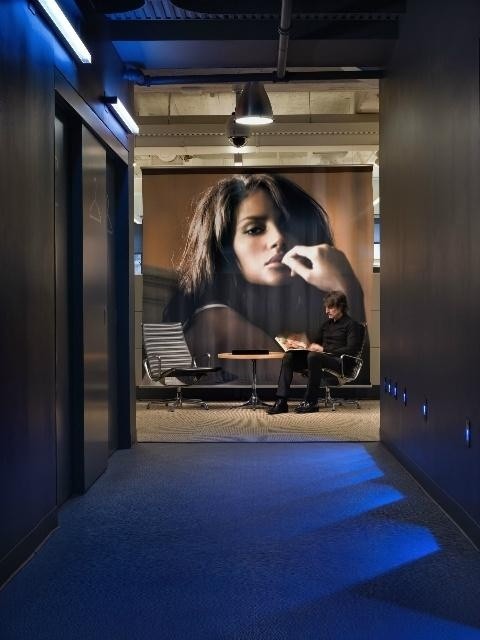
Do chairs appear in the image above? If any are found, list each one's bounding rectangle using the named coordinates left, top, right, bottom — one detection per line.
left=142, top=321, right=222, bottom=412
left=301, top=321, right=368, bottom=412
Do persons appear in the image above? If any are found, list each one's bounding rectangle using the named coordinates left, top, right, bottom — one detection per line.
left=263, top=288, right=364, bottom=415
left=156, top=174, right=369, bottom=384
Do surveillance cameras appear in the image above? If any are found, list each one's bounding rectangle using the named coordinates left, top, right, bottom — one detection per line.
left=226, top=114, right=251, bottom=148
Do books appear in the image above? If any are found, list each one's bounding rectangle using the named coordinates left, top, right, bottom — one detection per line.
left=274, top=331, right=335, bottom=357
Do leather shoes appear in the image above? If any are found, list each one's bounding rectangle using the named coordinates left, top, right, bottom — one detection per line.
left=266, top=401, right=290, bottom=415
left=293, top=399, right=321, bottom=414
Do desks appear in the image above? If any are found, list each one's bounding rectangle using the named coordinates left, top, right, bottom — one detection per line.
left=217, top=352, right=285, bottom=411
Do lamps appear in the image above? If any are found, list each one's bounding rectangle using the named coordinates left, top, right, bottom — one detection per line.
left=233, top=81, right=274, bottom=126
left=103, top=95, right=141, bottom=135
left=26, top=0, right=93, bottom=65
